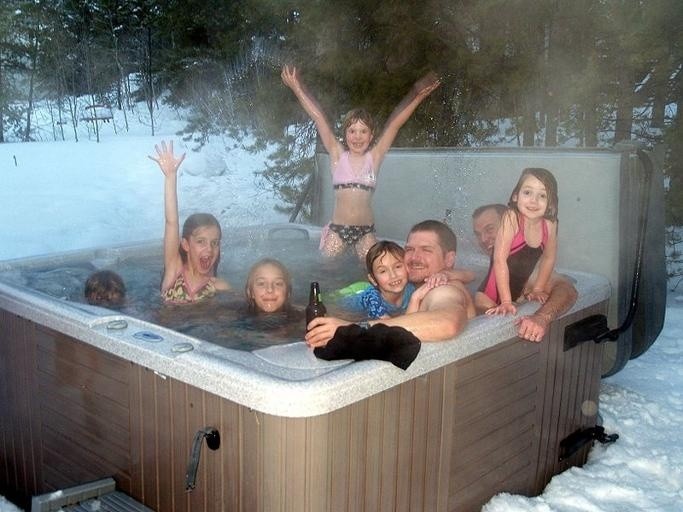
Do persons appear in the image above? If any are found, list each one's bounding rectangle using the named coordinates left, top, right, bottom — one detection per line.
left=474, top=168, right=558, bottom=318
left=338, top=240, right=476, bottom=320
left=245, top=258, right=306, bottom=314
left=472, top=204, right=578, bottom=342
left=281, top=65, right=442, bottom=259
left=148, top=139, right=231, bottom=305
left=304, top=220, right=476, bottom=349
left=84, top=271, right=126, bottom=310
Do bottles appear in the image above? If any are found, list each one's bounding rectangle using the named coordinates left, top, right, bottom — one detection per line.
left=305, top=280, right=327, bottom=335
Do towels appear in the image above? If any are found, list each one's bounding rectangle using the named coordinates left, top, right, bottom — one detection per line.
left=312, top=322, right=421, bottom=370
